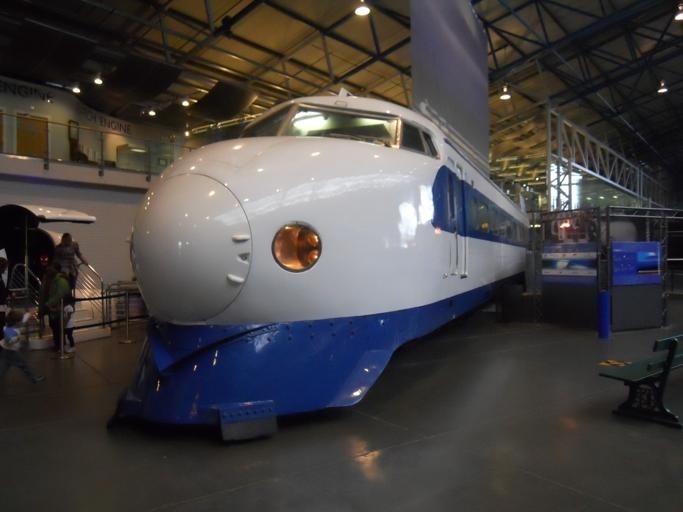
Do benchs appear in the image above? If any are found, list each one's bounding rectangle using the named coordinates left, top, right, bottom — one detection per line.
left=597, top=334, right=682, bottom=431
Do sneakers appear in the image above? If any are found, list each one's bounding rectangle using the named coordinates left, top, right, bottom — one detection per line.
left=64, top=345, right=76, bottom=353
left=33, top=375, right=46, bottom=384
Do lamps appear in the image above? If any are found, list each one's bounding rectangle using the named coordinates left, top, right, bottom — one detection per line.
left=70, top=69, right=106, bottom=95
left=351, top=0, right=370, bottom=19
left=675, top=0, right=683, bottom=24
left=498, top=84, right=512, bottom=104
left=656, top=78, right=669, bottom=96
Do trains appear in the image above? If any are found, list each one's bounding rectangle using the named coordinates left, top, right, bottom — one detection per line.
left=106, top=95, right=532, bottom=442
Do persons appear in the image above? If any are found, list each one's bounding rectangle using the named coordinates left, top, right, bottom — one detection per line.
left=52, top=234, right=88, bottom=288
left=39, top=262, right=72, bottom=351
left=0, top=257, right=16, bottom=352
left=0, top=309, right=46, bottom=385
left=55, top=294, right=76, bottom=353
